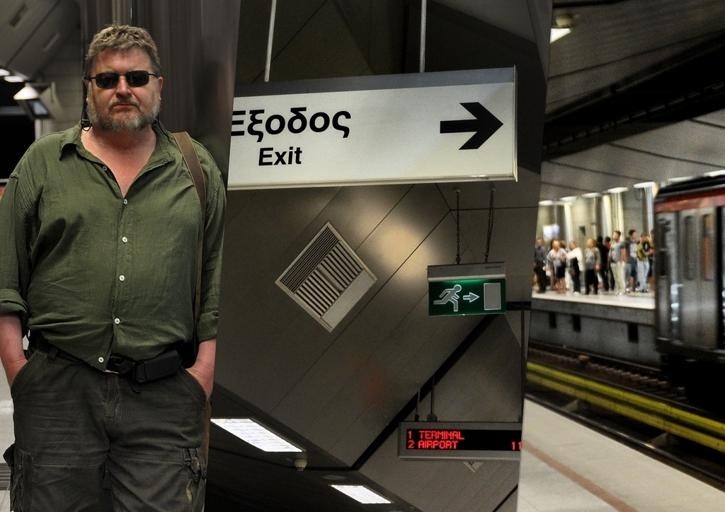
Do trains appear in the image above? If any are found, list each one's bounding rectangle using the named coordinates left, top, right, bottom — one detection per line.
left=653, top=174, right=724, bottom=374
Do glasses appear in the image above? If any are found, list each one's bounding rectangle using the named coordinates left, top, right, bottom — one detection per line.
left=86, top=69, right=158, bottom=90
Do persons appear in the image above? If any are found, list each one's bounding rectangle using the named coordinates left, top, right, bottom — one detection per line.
left=0, top=21, right=228, bottom=512
left=531, top=229, right=655, bottom=296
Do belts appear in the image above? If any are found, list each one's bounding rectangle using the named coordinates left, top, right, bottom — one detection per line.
left=32, top=339, right=181, bottom=376
